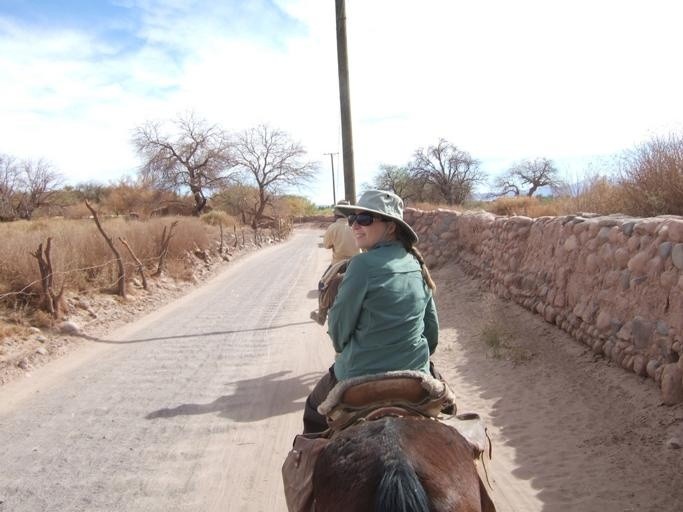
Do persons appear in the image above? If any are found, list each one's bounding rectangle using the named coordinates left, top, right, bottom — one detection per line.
left=310, top=200, right=367, bottom=325
left=302, top=190, right=458, bottom=434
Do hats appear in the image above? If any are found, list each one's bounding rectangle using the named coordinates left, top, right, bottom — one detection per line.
left=331, top=200, right=349, bottom=218
left=334, top=190, right=418, bottom=245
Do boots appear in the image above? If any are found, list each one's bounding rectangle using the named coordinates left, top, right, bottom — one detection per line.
left=311, top=291, right=327, bottom=325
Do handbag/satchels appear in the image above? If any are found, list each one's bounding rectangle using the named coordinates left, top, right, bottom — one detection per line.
left=282, top=428, right=336, bottom=512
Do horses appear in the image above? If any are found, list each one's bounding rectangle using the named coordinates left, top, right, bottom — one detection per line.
left=312, top=407, right=483, bottom=511
left=328, top=263, right=347, bottom=309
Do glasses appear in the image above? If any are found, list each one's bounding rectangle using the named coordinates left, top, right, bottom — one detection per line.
left=347, top=212, right=377, bottom=226
